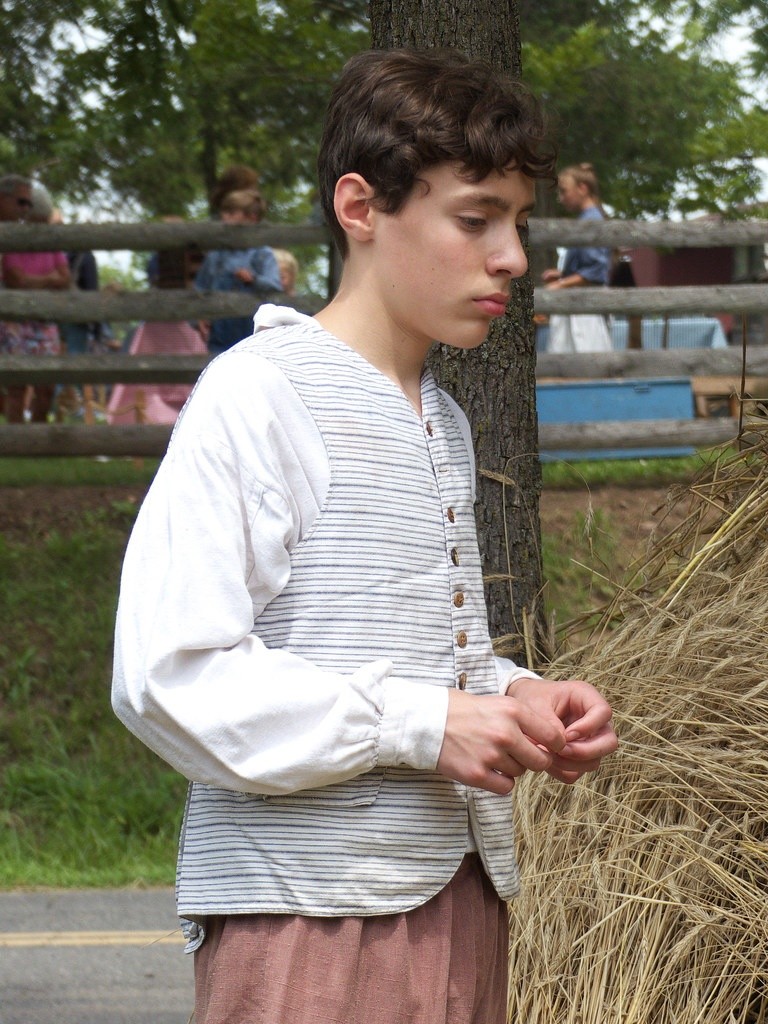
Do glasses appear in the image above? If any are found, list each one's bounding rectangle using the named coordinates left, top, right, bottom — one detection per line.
left=3, top=191, right=34, bottom=210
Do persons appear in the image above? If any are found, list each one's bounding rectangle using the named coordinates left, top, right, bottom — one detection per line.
left=0, top=166, right=297, bottom=423
left=544, top=161, right=614, bottom=351
left=112, top=48, right=618, bottom=1024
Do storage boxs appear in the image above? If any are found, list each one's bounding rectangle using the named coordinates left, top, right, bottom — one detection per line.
left=534, top=377, right=698, bottom=463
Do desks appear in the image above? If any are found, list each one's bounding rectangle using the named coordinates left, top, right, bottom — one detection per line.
left=537, top=317, right=727, bottom=417
